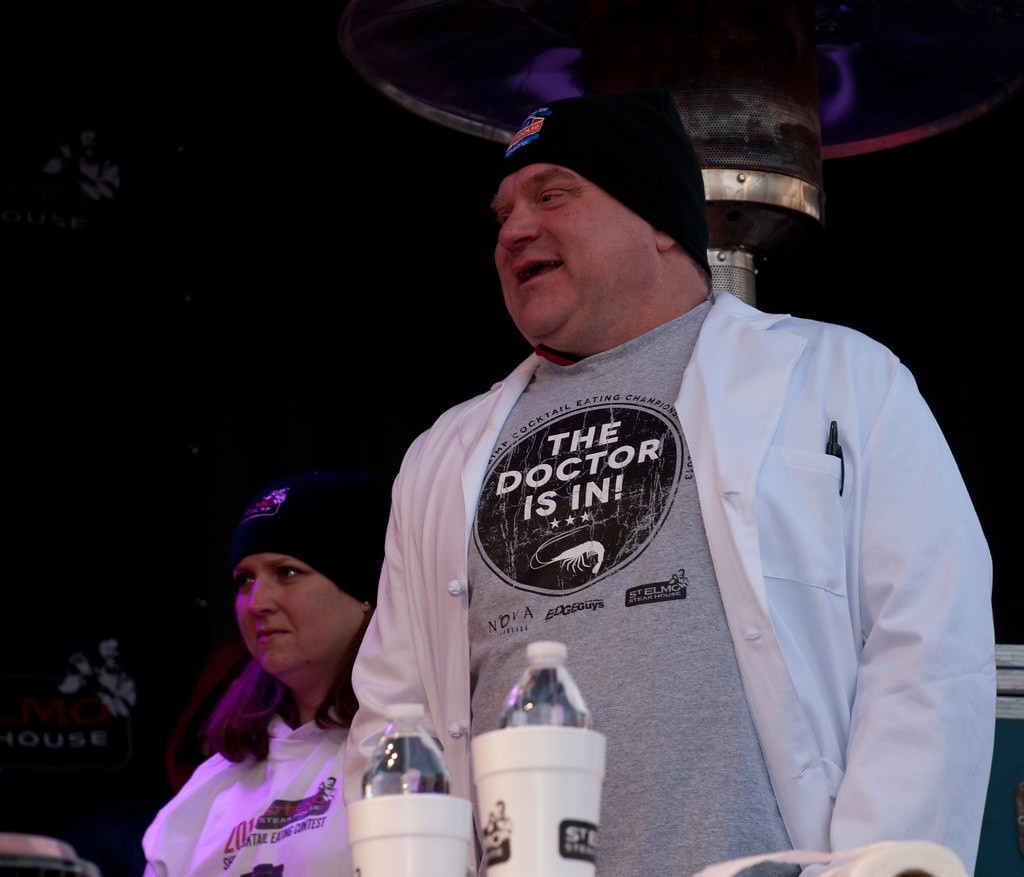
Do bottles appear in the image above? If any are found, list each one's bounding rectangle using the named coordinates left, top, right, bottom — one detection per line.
left=498, top=640, right=592, bottom=729
left=363, top=704, right=455, bottom=797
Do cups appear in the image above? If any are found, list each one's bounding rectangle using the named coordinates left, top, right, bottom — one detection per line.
left=468, top=726, right=610, bottom=877
left=347, top=793, right=472, bottom=877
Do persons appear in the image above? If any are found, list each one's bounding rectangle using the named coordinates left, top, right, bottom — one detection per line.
left=141, top=477, right=385, bottom=877
left=346, top=96, right=997, bottom=877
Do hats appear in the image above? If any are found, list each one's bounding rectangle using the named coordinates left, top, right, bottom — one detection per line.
left=227, top=486, right=380, bottom=606
left=494, top=86, right=715, bottom=282
